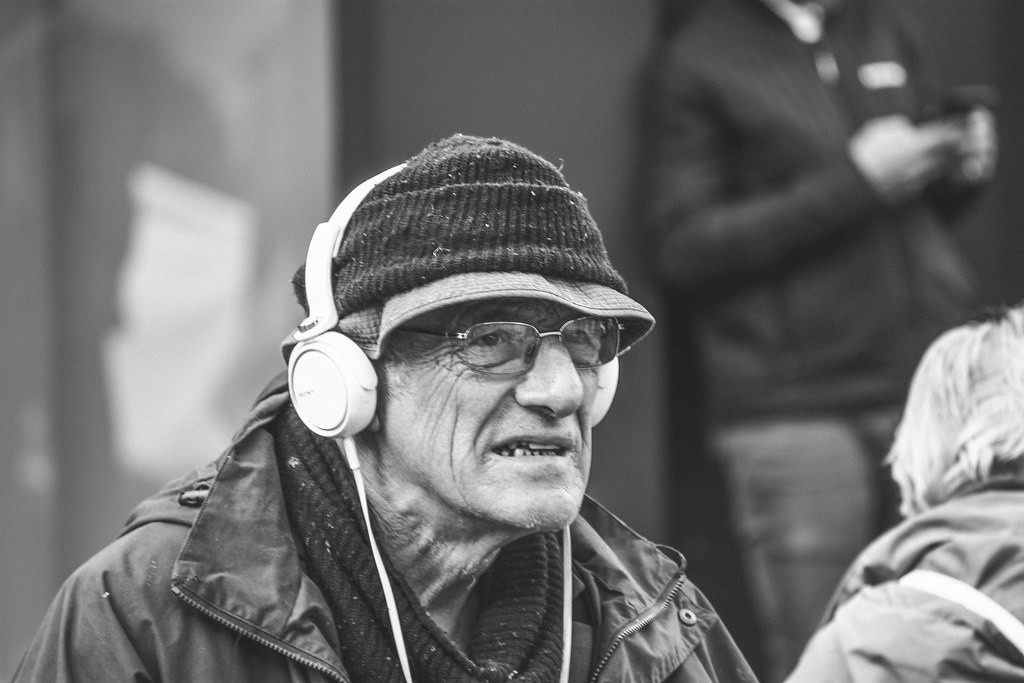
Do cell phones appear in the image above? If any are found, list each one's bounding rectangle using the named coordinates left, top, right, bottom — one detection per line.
left=936, top=84, right=992, bottom=121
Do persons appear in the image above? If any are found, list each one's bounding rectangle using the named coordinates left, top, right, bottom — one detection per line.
left=638, top=0, right=997, bottom=683
left=782, top=308, right=1024, bottom=683
left=12, top=136, right=758, bottom=683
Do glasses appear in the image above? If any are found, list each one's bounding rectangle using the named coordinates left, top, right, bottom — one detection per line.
left=396, top=313, right=623, bottom=376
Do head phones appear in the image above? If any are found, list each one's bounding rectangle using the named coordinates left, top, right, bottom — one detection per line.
left=286, top=164, right=621, bottom=437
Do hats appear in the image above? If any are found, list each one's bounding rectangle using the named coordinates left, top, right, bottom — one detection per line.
left=283, top=133, right=655, bottom=364
